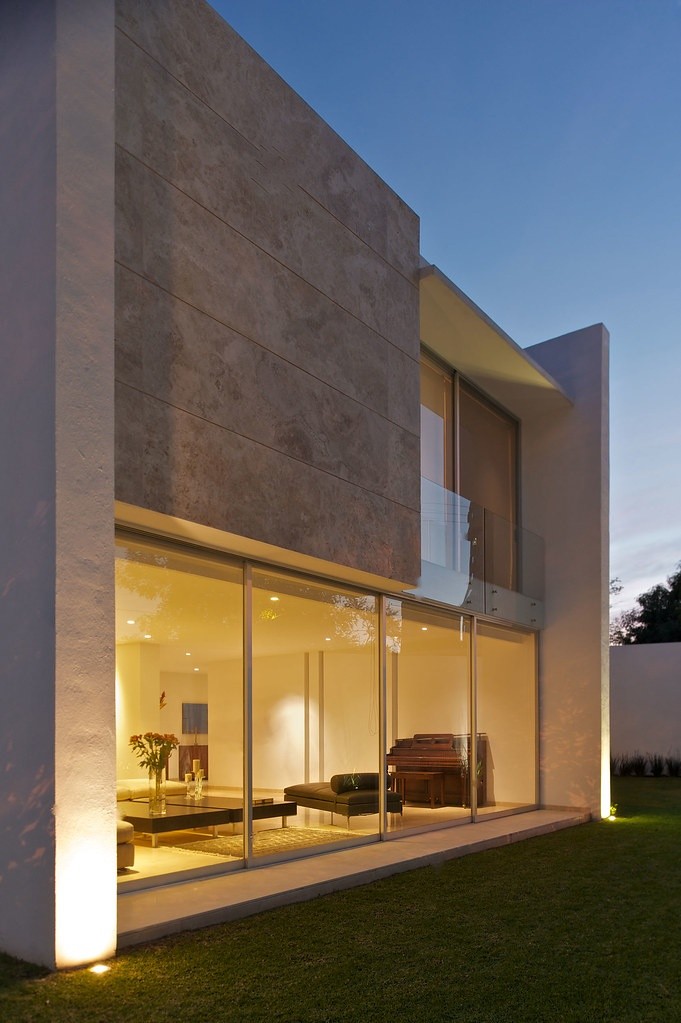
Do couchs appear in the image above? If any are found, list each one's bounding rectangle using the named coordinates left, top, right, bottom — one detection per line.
left=116, top=779, right=187, bottom=871
left=284, top=782, right=402, bottom=832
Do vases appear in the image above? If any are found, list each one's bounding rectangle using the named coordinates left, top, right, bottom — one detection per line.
left=148, top=764, right=166, bottom=815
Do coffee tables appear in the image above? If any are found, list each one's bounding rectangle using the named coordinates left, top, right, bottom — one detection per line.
left=117, top=801, right=229, bottom=848
left=133, top=793, right=297, bottom=837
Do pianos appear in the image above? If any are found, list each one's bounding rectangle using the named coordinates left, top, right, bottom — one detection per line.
left=386, top=738, right=487, bottom=809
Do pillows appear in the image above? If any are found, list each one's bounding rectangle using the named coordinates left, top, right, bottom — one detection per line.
left=331, top=773, right=393, bottom=794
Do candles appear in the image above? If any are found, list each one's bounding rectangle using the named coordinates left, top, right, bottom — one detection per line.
left=184, top=760, right=205, bottom=780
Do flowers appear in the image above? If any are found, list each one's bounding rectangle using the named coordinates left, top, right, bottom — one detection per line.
left=130, top=733, right=180, bottom=791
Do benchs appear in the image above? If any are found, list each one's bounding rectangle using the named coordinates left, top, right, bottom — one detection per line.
left=390, top=769, right=446, bottom=809
